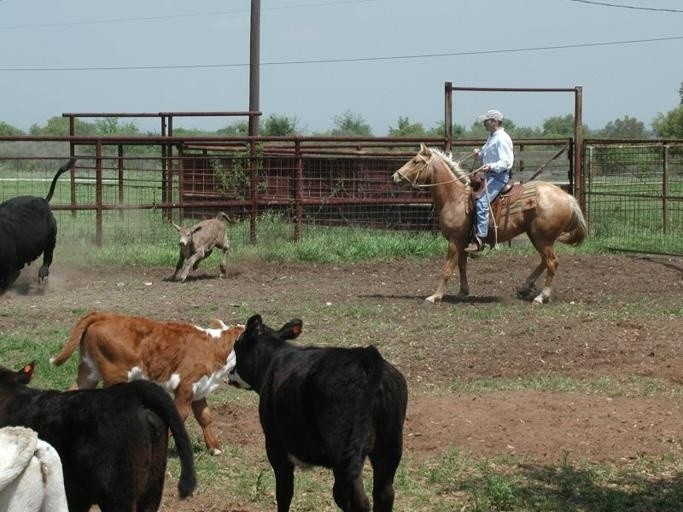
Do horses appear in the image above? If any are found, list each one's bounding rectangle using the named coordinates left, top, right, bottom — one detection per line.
left=390, top=142, right=589, bottom=306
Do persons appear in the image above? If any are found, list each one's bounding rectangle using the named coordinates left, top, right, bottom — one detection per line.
left=462, top=110, right=517, bottom=256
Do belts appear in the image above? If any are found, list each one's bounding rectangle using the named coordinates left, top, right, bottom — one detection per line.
left=490, top=170, right=506, bottom=173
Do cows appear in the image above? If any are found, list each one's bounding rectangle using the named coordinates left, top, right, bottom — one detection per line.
left=0, top=359, right=196, bottom=512
left=168, top=211, right=232, bottom=284
left=49, top=311, right=247, bottom=457
left=227, top=314, right=408, bottom=512
left=0, top=157, right=79, bottom=295
left=0, top=426, right=69, bottom=511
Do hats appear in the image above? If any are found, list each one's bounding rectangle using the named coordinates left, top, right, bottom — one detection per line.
left=479, top=111, right=503, bottom=121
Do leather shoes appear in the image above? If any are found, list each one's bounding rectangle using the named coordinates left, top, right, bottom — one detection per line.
left=464, top=241, right=484, bottom=251
